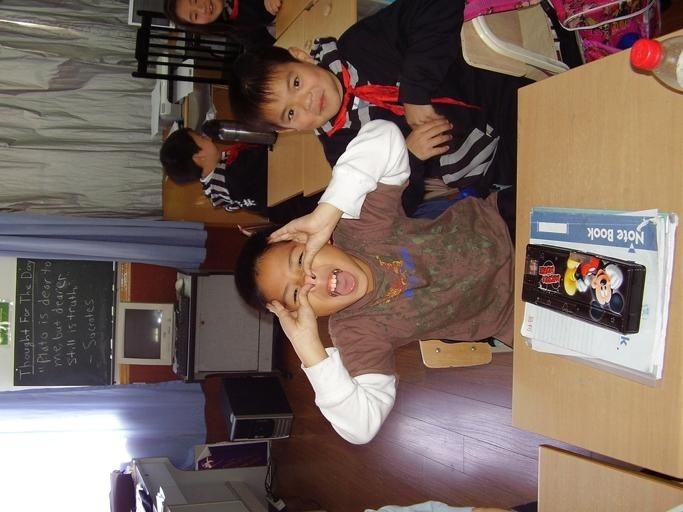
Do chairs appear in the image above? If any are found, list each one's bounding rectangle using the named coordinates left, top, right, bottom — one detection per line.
left=458, top=0, right=571, bottom=82
left=417, top=338, right=513, bottom=369
left=131, top=10, right=246, bottom=85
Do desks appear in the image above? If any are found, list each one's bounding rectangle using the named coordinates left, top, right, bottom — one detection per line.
left=265, top=0, right=390, bottom=208
left=130, top=456, right=271, bottom=512
left=180, top=28, right=236, bottom=128
left=176, top=270, right=292, bottom=383
